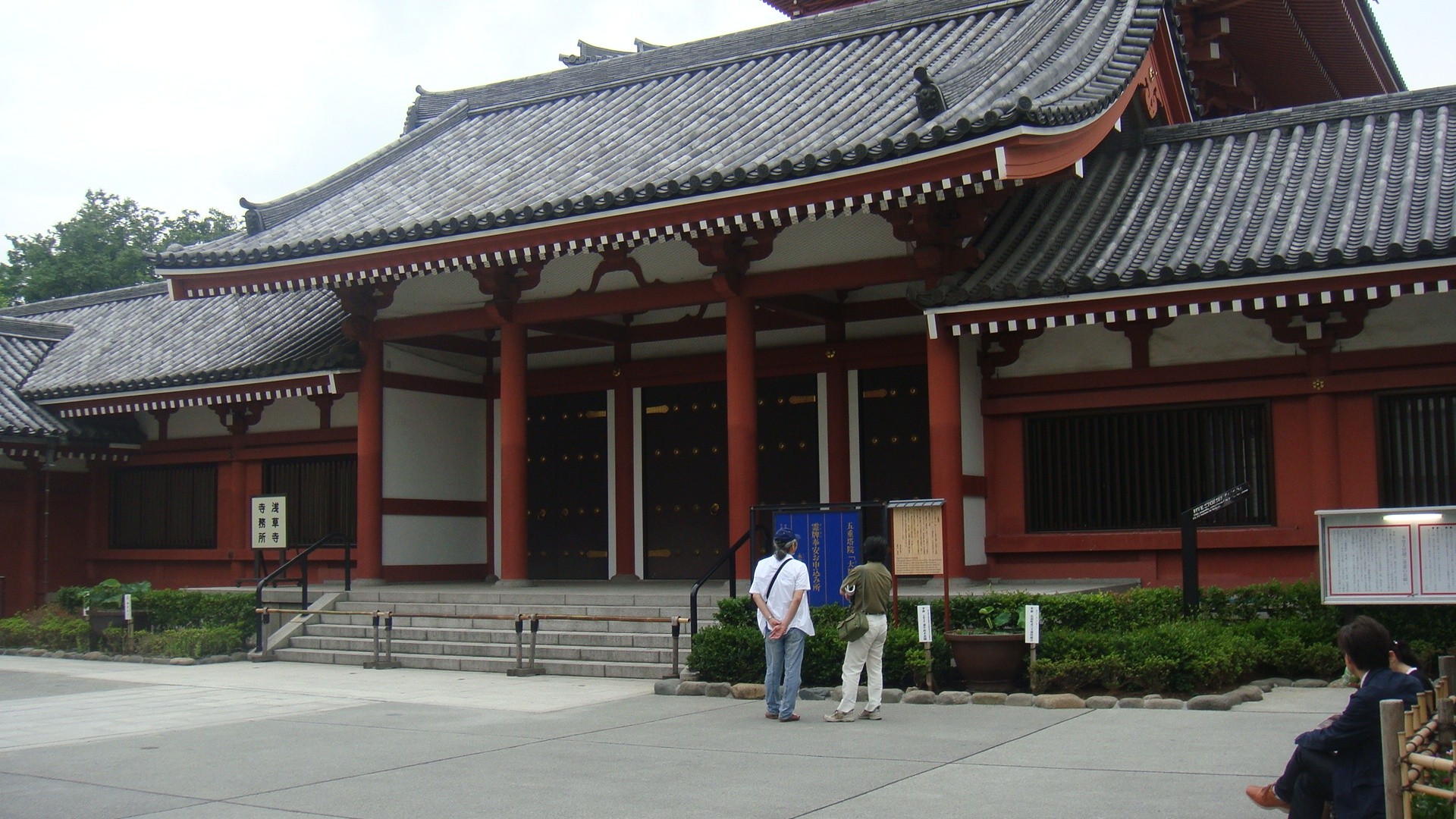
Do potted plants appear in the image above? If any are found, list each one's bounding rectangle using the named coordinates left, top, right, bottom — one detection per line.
left=943, top=605, right=1028, bottom=692
left=83, top=578, right=153, bottom=652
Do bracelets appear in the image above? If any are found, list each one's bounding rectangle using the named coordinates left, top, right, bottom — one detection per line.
left=767, top=616, right=772, bottom=620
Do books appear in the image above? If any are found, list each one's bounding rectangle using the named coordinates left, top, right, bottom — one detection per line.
left=844, top=585, right=855, bottom=604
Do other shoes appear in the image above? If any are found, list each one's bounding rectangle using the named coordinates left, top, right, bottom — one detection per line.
left=765, top=712, right=778, bottom=719
left=779, top=714, right=800, bottom=722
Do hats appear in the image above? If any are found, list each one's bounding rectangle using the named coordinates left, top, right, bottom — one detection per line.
left=774, top=528, right=800, bottom=544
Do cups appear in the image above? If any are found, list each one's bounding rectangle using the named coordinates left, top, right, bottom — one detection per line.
left=1343, top=667, right=1361, bottom=686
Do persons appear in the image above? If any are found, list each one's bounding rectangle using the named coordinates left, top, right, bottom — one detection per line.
left=824, top=535, right=893, bottom=722
left=749, top=528, right=815, bottom=722
left=1245, top=615, right=1435, bottom=819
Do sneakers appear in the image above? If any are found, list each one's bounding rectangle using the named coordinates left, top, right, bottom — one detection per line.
left=1246, top=782, right=1292, bottom=814
left=859, top=706, right=882, bottom=720
left=824, top=709, right=855, bottom=721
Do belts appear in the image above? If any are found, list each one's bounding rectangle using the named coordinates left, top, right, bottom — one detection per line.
left=865, top=612, right=884, bottom=614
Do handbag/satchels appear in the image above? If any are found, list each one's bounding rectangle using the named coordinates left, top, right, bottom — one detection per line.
left=836, top=612, right=870, bottom=642
